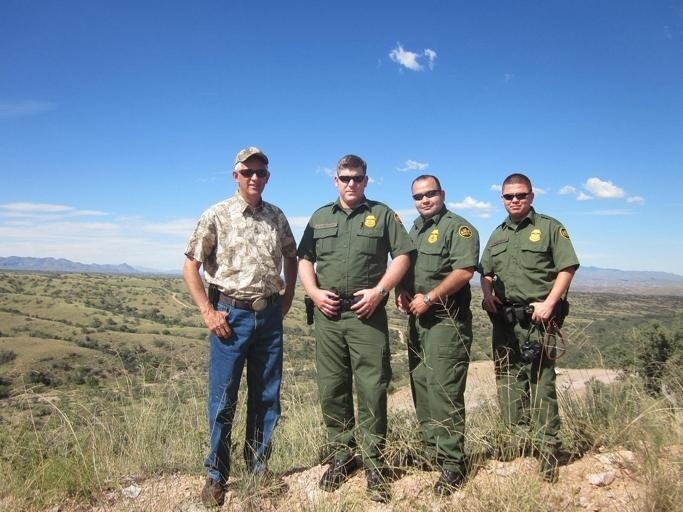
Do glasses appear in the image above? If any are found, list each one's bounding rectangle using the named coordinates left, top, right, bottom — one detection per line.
left=238, top=169, right=268, bottom=178
left=412, top=190, right=441, bottom=201
left=503, top=193, right=530, bottom=199
left=337, top=173, right=365, bottom=182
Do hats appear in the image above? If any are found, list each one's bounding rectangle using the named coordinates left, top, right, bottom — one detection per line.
left=235, top=147, right=268, bottom=166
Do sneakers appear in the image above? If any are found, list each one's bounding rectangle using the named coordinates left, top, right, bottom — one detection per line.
left=434, top=469, right=471, bottom=495
left=201, top=477, right=225, bottom=507
left=539, top=453, right=559, bottom=482
left=408, top=455, right=442, bottom=471
left=365, top=468, right=390, bottom=504
left=319, top=458, right=356, bottom=492
left=486, top=443, right=526, bottom=462
left=251, top=468, right=281, bottom=483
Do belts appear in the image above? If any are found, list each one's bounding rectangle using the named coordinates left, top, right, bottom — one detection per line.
left=217, top=293, right=282, bottom=311
left=336, top=297, right=364, bottom=310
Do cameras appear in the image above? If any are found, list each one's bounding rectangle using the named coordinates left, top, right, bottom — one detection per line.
left=521, top=342, right=544, bottom=362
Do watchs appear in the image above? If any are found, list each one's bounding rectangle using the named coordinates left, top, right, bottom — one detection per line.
left=421, top=293, right=434, bottom=307
left=372, top=284, right=389, bottom=298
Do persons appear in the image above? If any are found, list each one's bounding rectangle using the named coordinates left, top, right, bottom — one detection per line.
left=296, top=155, right=418, bottom=502
left=182, top=147, right=298, bottom=508
left=394, top=176, right=478, bottom=496
left=474, top=172, right=581, bottom=463
